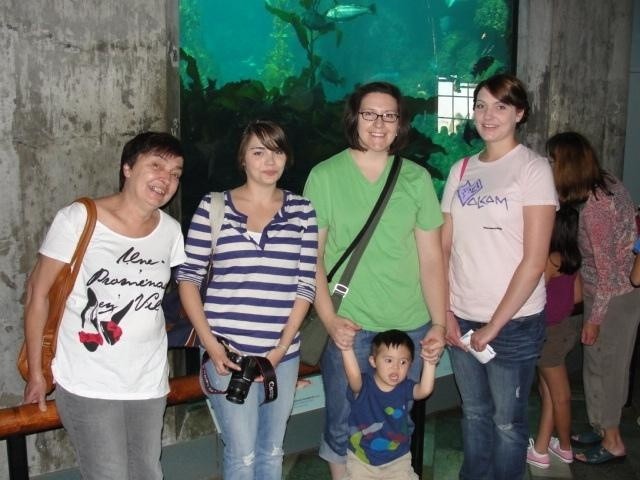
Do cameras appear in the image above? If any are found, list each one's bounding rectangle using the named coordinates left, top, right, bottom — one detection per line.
left=202, top=335, right=278, bottom=407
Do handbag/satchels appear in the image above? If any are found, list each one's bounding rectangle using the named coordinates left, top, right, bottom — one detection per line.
left=17, top=195, right=98, bottom=394
left=161, top=192, right=226, bottom=347
left=298, top=154, right=403, bottom=368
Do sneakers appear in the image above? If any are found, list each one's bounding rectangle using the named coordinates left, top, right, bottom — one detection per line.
left=548, top=436, right=575, bottom=465
left=522, top=438, right=551, bottom=469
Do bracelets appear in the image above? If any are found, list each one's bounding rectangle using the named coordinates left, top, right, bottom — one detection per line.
left=275, top=344, right=288, bottom=352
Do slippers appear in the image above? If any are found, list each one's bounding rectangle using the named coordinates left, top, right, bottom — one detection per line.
left=570, top=429, right=606, bottom=446
left=572, top=442, right=627, bottom=465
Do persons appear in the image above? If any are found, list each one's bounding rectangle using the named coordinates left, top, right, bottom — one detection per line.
left=440, top=75, right=560, bottom=479
left=526, top=205, right=583, bottom=469
left=630, top=215, right=640, bottom=287
left=175, top=120, right=318, bottom=480
left=302, top=83, right=447, bottom=480
left=333, top=325, right=442, bottom=480
left=546, top=132, right=639, bottom=468
left=23, top=131, right=188, bottom=479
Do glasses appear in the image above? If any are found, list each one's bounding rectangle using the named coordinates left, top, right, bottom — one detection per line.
left=354, top=111, right=402, bottom=123
